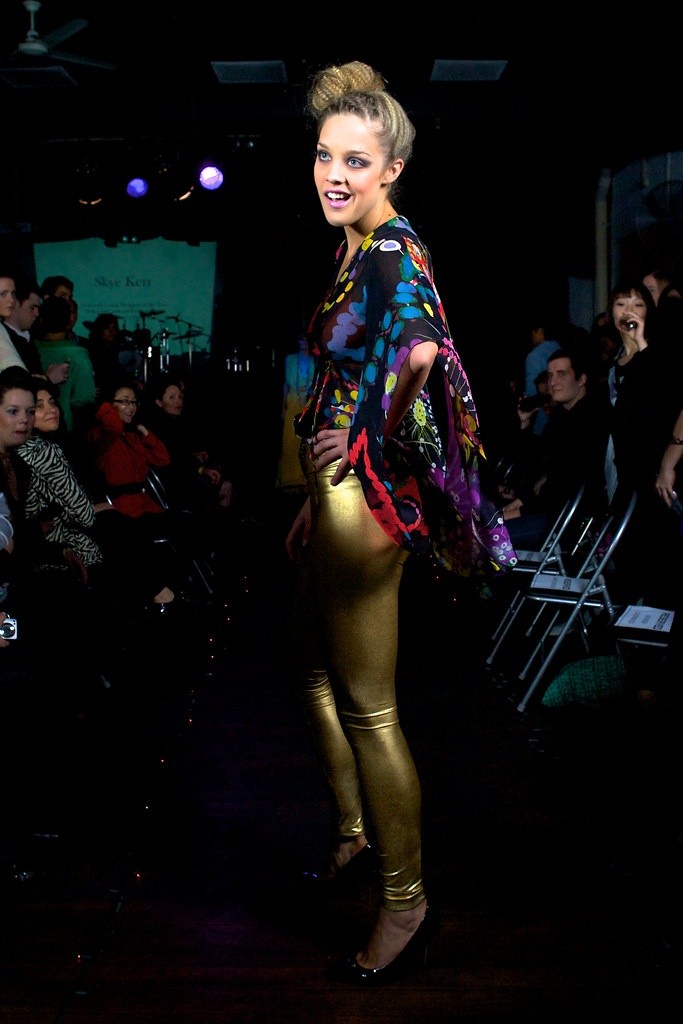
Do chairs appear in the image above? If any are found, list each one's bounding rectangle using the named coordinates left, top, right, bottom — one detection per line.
left=138, top=436, right=656, bottom=709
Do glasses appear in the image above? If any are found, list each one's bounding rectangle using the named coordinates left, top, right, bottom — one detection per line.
left=111, top=398, right=139, bottom=407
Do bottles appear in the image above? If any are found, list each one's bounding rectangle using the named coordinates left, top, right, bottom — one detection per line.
left=224, top=344, right=251, bottom=377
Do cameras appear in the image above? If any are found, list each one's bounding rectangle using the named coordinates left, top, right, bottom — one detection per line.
left=0, top=618, right=17, bottom=640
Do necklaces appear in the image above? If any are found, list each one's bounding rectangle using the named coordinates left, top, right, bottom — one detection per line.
left=295, top=353, right=310, bottom=400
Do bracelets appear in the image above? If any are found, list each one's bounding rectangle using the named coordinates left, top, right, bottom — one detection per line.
left=666, top=434, right=683, bottom=447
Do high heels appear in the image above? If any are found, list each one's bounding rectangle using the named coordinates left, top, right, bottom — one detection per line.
left=302, top=844, right=384, bottom=899
left=327, top=903, right=440, bottom=982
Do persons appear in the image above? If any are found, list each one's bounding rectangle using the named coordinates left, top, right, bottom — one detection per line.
left=279, top=59, right=524, bottom=1002
left=0, top=271, right=234, bottom=708
left=463, top=259, right=683, bottom=723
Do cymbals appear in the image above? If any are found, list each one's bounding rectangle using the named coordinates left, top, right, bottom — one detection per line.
left=142, top=308, right=167, bottom=318
left=172, top=332, right=203, bottom=340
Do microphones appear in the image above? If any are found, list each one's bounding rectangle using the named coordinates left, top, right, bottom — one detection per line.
left=150, top=311, right=156, bottom=319
